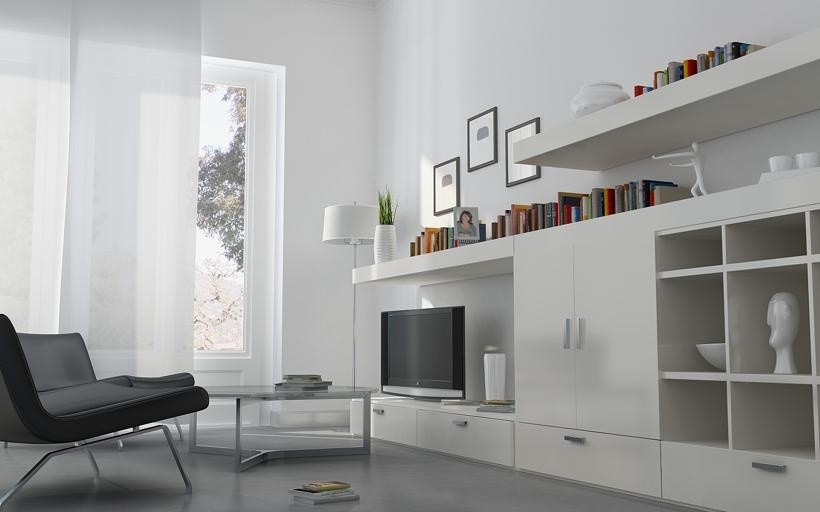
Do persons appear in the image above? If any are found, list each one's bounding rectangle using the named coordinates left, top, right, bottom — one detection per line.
left=457, top=211, right=477, bottom=237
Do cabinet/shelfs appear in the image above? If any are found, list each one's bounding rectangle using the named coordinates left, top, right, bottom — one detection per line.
left=516, top=204, right=660, bottom=500
left=348, top=236, right=515, bottom=474
left=656, top=168, right=820, bottom=512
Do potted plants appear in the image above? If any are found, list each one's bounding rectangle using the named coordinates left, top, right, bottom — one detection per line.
left=373, top=184, right=399, bottom=263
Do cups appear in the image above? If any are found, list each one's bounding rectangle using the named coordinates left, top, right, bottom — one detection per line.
left=794, top=152, right=819, bottom=169
left=768, top=155, right=793, bottom=172
left=483, top=351, right=507, bottom=402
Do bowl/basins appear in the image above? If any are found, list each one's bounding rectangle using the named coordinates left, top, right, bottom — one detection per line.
left=694, top=342, right=726, bottom=372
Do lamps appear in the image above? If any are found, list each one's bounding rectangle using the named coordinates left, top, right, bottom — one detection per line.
left=320, top=202, right=380, bottom=389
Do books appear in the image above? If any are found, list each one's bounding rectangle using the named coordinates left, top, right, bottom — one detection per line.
left=491, top=178, right=689, bottom=240
left=456, top=224, right=486, bottom=248
left=634, top=42, right=767, bottom=97
left=273, top=374, right=332, bottom=393
left=410, top=226, right=454, bottom=258
left=288, top=481, right=360, bottom=508
left=453, top=206, right=480, bottom=241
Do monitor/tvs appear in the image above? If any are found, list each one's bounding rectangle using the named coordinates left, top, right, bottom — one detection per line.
left=380, top=304, right=467, bottom=400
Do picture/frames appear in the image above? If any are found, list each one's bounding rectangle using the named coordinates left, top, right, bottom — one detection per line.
left=432, top=156, right=460, bottom=216
left=466, top=106, right=498, bottom=173
left=504, top=117, right=541, bottom=188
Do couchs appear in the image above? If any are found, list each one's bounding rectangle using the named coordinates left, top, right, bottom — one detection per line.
left=0, top=312, right=209, bottom=512
left=18, top=331, right=195, bottom=450
